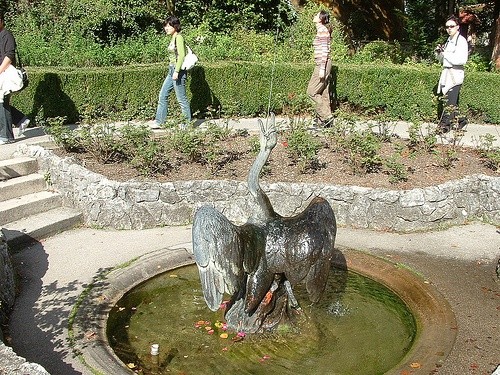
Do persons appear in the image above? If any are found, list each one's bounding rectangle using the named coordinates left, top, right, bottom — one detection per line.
left=0, top=10, right=30, bottom=145
left=435, top=16, right=468, bottom=134
left=306, top=10, right=334, bottom=129
left=147, top=16, right=192, bottom=129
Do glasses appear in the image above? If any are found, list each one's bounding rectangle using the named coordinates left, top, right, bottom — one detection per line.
left=445, top=26, right=454, bottom=28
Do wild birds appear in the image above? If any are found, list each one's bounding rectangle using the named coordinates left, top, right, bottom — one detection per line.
left=192, top=112, right=337, bottom=315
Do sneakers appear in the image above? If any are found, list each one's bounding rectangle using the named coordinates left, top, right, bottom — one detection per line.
left=18, top=119, right=30, bottom=136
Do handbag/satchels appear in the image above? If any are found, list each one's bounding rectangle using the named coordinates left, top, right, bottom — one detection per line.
left=17, top=68, right=28, bottom=91
left=175, top=34, right=198, bottom=71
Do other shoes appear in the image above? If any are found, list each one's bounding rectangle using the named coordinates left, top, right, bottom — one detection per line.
left=453, top=119, right=468, bottom=129
left=321, top=117, right=334, bottom=126
left=150, top=124, right=161, bottom=128
left=0, top=141, right=15, bottom=144
